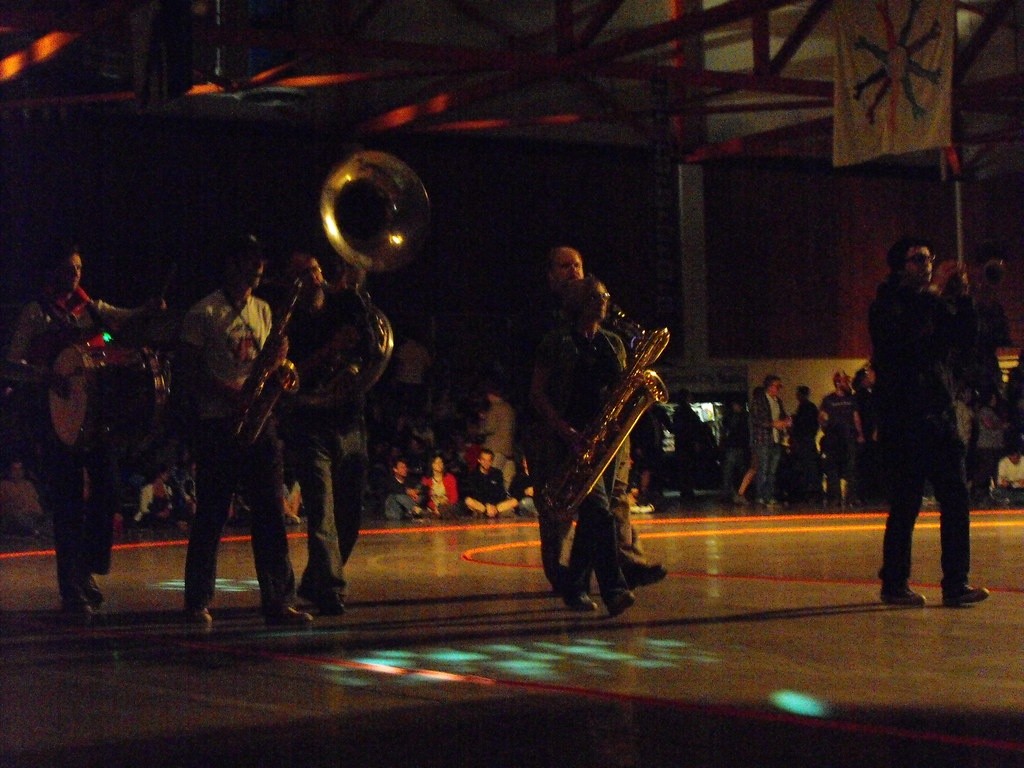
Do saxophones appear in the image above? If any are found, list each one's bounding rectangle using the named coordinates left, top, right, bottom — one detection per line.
left=540, top=307, right=670, bottom=524
left=236, top=267, right=300, bottom=443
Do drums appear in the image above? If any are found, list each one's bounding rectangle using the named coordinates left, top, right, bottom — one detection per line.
left=48, top=343, right=171, bottom=450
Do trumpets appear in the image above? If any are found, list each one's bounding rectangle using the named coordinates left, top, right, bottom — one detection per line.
left=930, top=264, right=982, bottom=299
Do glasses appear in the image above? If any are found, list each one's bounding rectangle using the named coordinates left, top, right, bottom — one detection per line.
left=905, top=252, right=937, bottom=265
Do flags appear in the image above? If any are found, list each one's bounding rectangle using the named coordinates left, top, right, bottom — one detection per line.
left=832, top=0, right=950, bottom=164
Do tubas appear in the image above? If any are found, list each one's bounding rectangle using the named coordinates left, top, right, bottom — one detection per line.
left=291, top=154, right=430, bottom=407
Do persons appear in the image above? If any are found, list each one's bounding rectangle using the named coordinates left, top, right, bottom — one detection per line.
left=1, top=240, right=166, bottom=619
left=867, top=236, right=988, bottom=605
left=1, top=345, right=1023, bottom=541
left=538, top=245, right=668, bottom=594
left=531, top=274, right=635, bottom=618
left=177, top=229, right=315, bottom=629
left=267, top=242, right=370, bottom=617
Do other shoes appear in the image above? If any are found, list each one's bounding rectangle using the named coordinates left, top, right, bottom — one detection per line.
left=62, top=597, right=92, bottom=613
left=319, top=591, right=347, bottom=616
left=264, top=604, right=314, bottom=626
left=83, top=576, right=104, bottom=604
left=563, top=592, right=598, bottom=611
left=880, top=587, right=925, bottom=606
left=942, top=584, right=988, bottom=607
left=184, top=607, right=212, bottom=623
left=296, top=582, right=345, bottom=605
left=606, top=591, right=635, bottom=619
left=622, top=560, right=666, bottom=590
